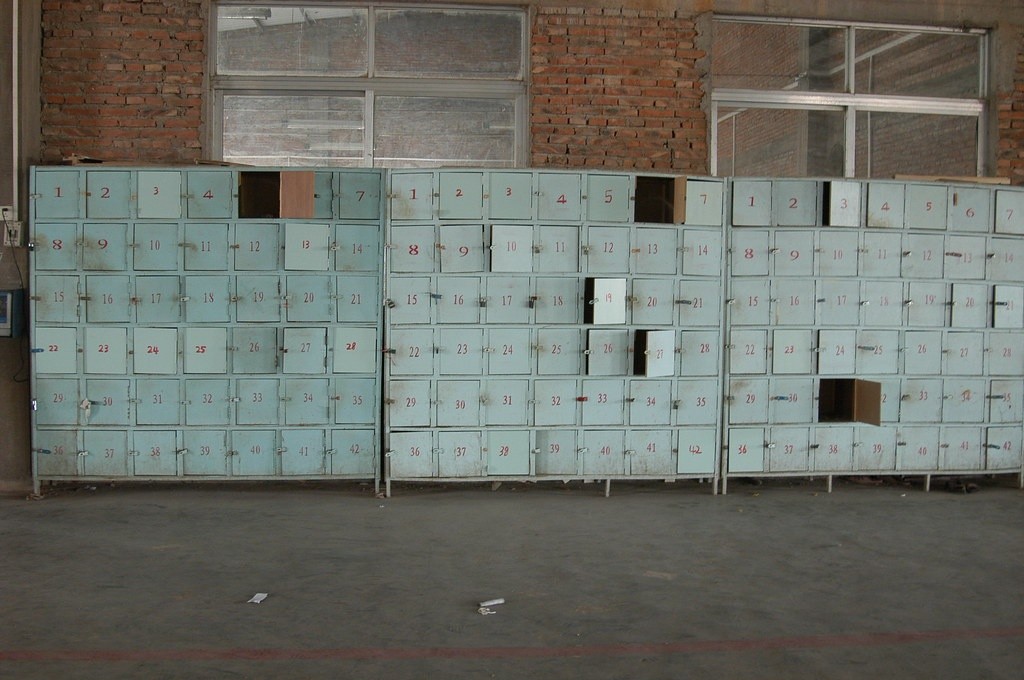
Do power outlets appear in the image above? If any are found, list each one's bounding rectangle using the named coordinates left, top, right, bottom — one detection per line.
left=0, top=206, right=14, bottom=220
left=4, top=221, right=23, bottom=247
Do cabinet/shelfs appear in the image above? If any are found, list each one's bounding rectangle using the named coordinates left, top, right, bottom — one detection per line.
left=386, top=169, right=727, bottom=497
left=28, top=164, right=386, bottom=499
left=721, top=176, right=1024, bottom=497
